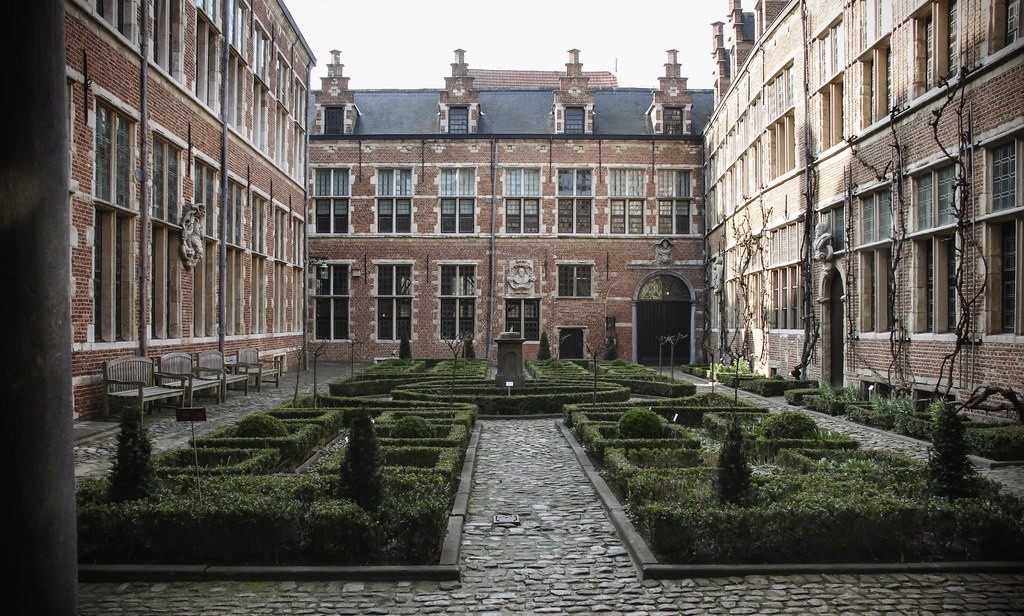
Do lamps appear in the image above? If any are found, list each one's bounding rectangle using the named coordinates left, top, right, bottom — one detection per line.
left=319, top=264, right=329, bottom=280
left=352, top=267, right=361, bottom=277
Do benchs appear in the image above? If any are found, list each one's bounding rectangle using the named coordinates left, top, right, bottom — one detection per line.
left=101, top=347, right=283, bottom=420
